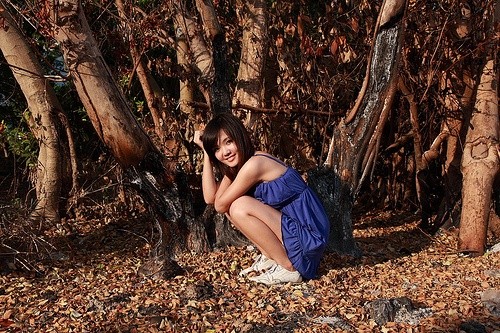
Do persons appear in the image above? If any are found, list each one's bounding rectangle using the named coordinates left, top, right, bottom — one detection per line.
left=193, top=111, right=332, bottom=286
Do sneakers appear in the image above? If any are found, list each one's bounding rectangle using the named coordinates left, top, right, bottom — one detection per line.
left=253, top=264, right=302, bottom=285
left=238, top=255, right=275, bottom=277
